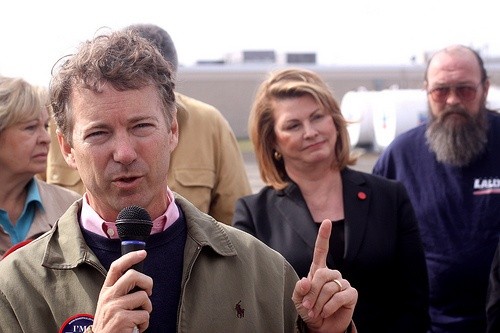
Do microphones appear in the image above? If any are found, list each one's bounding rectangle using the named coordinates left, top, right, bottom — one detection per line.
left=114, top=205, right=153, bottom=333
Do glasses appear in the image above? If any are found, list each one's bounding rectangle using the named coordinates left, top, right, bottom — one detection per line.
left=427, top=82, right=482, bottom=103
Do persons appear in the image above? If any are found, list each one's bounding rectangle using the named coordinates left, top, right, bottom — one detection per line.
left=0, top=29, right=360, bottom=332
left=0, top=75, right=82, bottom=259
left=369, top=43, right=499, bottom=333
left=40, top=23, right=253, bottom=231
left=231, top=63, right=430, bottom=333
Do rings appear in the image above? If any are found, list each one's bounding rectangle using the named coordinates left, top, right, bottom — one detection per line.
left=331, top=278, right=343, bottom=291
